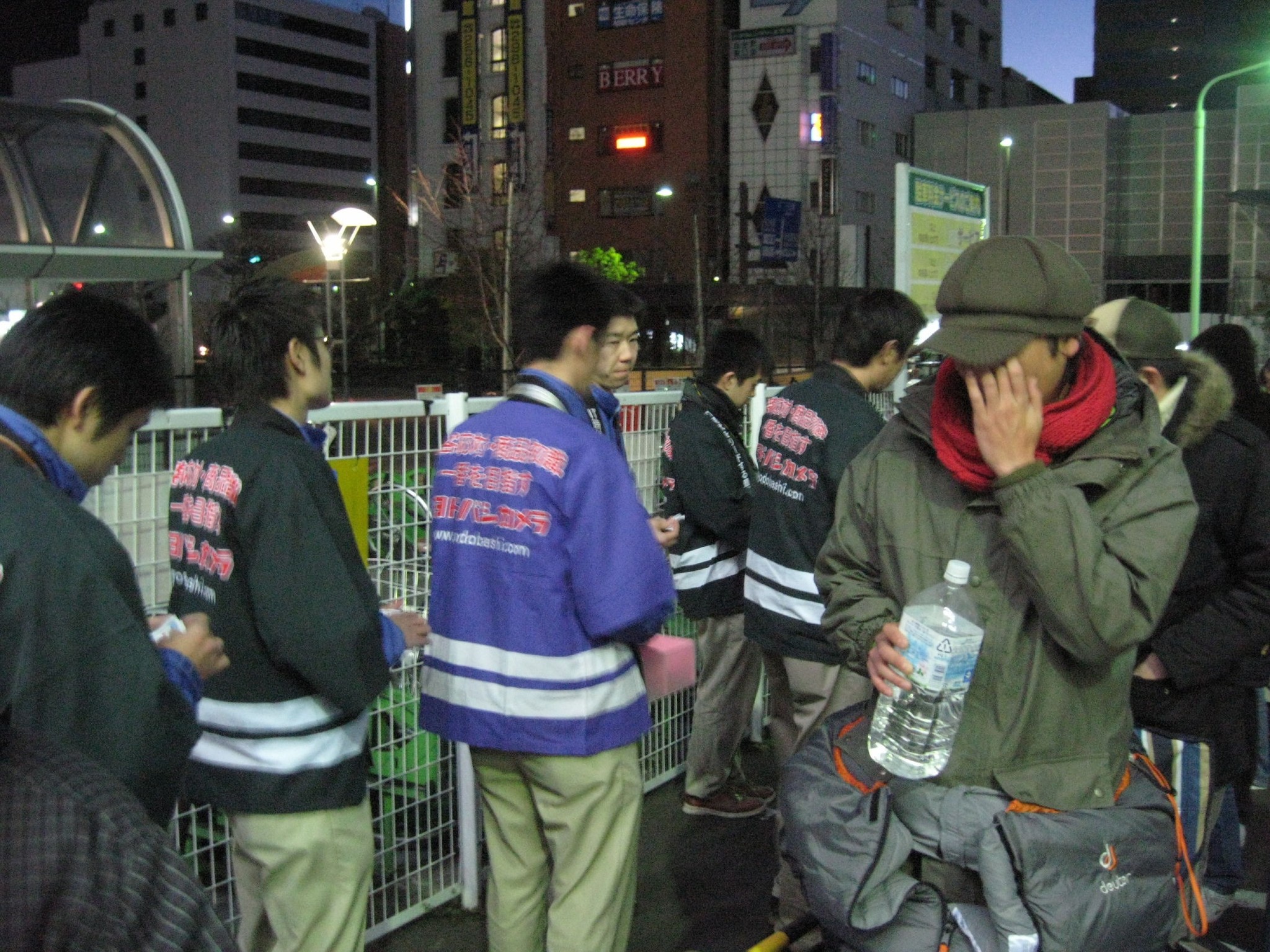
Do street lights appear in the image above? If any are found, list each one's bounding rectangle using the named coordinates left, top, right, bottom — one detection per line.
left=307, top=207, right=378, bottom=402
left=655, top=184, right=707, bottom=363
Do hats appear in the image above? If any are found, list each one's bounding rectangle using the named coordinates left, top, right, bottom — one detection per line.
left=909, top=233, right=1095, bottom=369
left=1083, top=295, right=1185, bottom=359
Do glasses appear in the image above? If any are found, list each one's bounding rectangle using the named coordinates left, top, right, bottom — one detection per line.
left=312, top=332, right=332, bottom=346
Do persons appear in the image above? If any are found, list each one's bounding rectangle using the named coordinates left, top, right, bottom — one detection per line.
left=0, top=291, right=230, bottom=952
left=417, top=257, right=675, bottom=952
left=582, top=287, right=680, bottom=546
left=167, top=278, right=432, bottom=951
left=659, top=234, right=1269, bottom=952
left=0, top=726, right=241, bottom=952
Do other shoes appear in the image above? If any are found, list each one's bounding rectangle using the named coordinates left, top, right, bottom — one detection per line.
left=1249, top=784, right=1268, bottom=790
left=727, top=773, right=776, bottom=803
left=1198, top=886, right=1237, bottom=924
left=682, top=787, right=767, bottom=817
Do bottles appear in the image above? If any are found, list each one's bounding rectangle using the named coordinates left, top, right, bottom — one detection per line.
left=866, top=558, right=984, bottom=779
